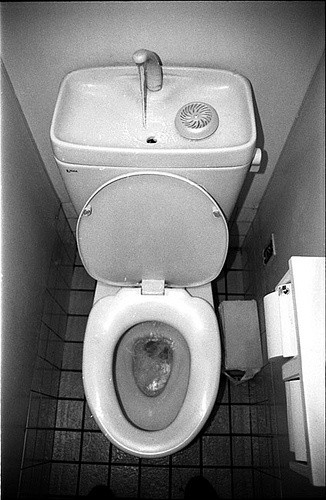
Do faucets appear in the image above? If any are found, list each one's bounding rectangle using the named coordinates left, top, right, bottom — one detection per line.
left=132, top=50, right=163, bottom=92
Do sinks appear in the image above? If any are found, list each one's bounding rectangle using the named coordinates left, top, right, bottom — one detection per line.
left=50, top=67, right=252, bottom=148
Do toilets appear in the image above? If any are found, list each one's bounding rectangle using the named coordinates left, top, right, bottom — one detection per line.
left=49, top=64, right=264, bottom=459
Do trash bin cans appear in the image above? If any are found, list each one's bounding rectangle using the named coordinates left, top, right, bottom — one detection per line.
left=217, top=298, right=265, bottom=385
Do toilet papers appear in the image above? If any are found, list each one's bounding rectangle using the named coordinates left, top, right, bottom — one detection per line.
left=264, top=284, right=298, bottom=363
left=284, top=379, right=310, bottom=462
